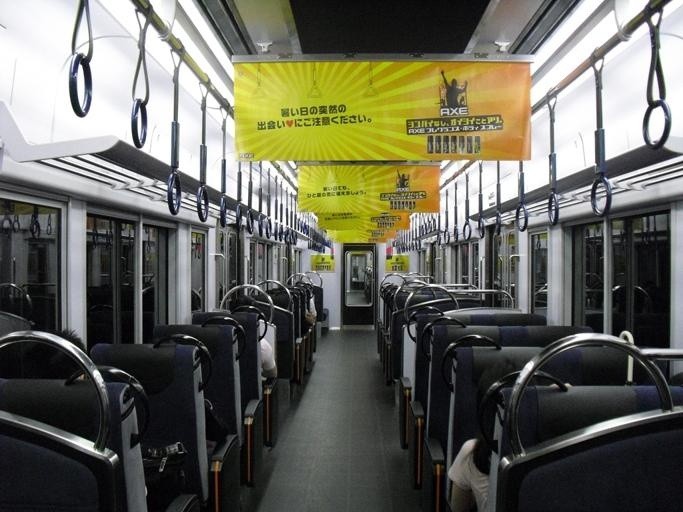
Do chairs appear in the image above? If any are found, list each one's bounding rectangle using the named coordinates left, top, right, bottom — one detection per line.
left=1, top=272, right=330, bottom=512
left=375, top=270, right=682, bottom=509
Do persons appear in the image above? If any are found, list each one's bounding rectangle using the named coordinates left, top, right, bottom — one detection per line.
left=305, top=293, right=317, bottom=324
left=445, top=358, right=520, bottom=512
left=230, top=293, right=278, bottom=382
left=23, top=329, right=88, bottom=380
